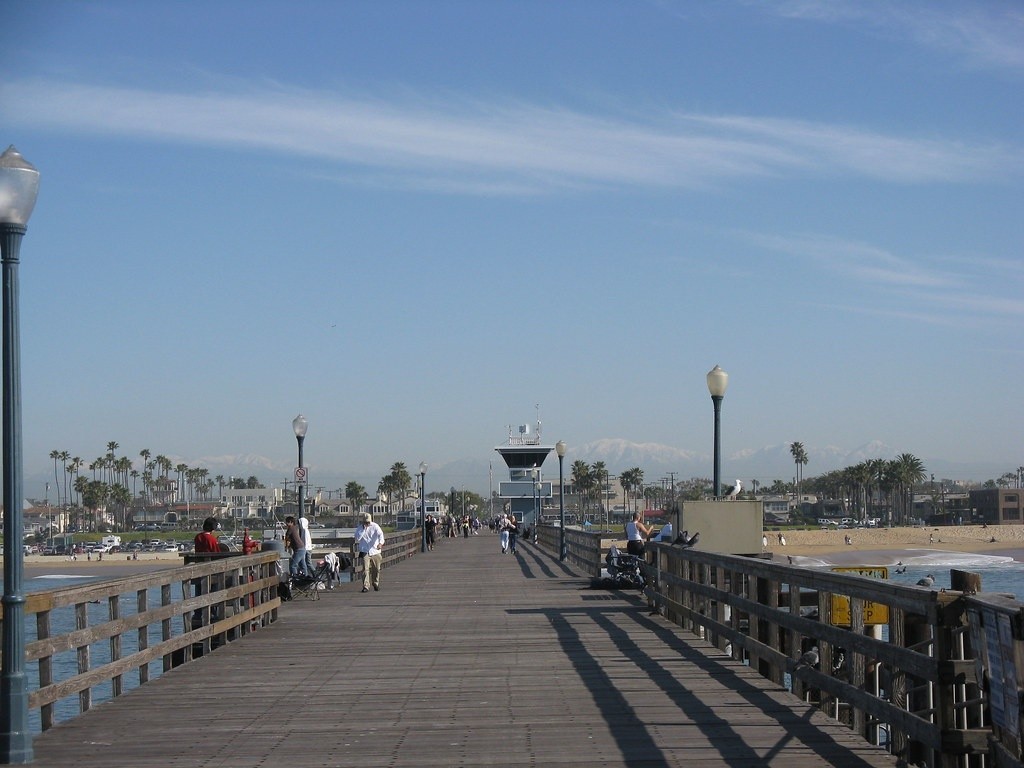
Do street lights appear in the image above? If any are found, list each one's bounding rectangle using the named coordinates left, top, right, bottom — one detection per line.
left=531, top=468, right=538, bottom=545
left=293, top=414, right=309, bottom=539
left=419, top=461, right=428, bottom=553
left=0, top=143, right=41, bottom=768
left=536, top=482, right=542, bottom=515
left=555, top=440, right=567, bottom=562
left=707, top=364, right=729, bottom=499
left=450, top=486, right=454, bottom=515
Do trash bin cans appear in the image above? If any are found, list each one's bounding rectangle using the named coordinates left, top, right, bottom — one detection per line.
left=262, top=540, right=291, bottom=576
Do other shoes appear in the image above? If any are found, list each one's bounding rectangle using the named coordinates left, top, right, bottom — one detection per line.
left=502, top=548, right=505, bottom=553
left=427, top=545, right=430, bottom=551
left=361, top=587, right=370, bottom=593
left=430, top=545, right=434, bottom=551
left=372, top=582, right=379, bottom=591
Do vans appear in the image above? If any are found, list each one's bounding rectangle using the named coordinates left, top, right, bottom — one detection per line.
left=160, top=523, right=179, bottom=532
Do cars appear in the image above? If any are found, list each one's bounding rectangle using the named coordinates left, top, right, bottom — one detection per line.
left=860, top=517, right=881, bottom=525
left=818, top=519, right=836, bottom=526
left=308, top=521, right=326, bottom=529
left=0, top=537, right=252, bottom=554
left=841, top=518, right=858, bottom=525
left=276, top=522, right=287, bottom=528
left=136, top=522, right=161, bottom=532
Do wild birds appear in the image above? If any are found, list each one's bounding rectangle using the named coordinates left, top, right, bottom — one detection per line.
left=919, top=573, right=935, bottom=587
left=671, top=530, right=700, bottom=550
left=724, top=479, right=742, bottom=497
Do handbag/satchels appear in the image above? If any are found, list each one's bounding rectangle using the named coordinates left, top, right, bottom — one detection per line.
left=515, top=521, right=520, bottom=535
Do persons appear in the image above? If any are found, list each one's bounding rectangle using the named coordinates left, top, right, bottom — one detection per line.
left=498, top=513, right=521, bottom=555
left=624, top=512, right=654, bottom=585
left=447, top=512, right=479, bottom=538
left=425, top=514, right=436, bottom=551
left=488, top=515, right=500, bottom=534
left=845, top=534, right=852, bottom=545
left=285, top=516, right=322, bottom=588
left=87, top=551, right=91, bottom=561
left=778, top=532, right=786, bottom=546
left=929, top=533, right=934, bottom=544
left=72, top=543, right=76, bottom=554
left=191, top=517, right=222, bottom=621
left=355, top=513, right=385, bottom=592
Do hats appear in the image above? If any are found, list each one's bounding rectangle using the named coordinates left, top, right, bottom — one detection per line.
left=363, top=513, right=371, bottom=522
